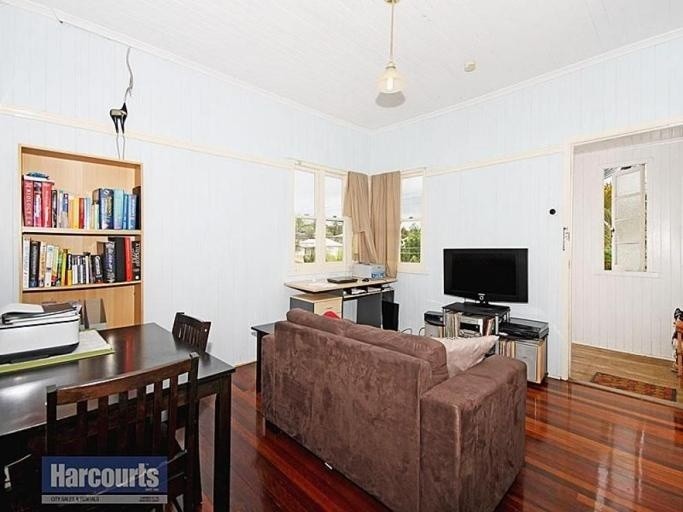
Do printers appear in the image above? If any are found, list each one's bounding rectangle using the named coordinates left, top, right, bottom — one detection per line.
left=0, top=303, right=81, bottom=365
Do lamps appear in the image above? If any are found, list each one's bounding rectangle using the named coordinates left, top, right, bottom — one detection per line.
left=377, top=0, right=403, bottom=94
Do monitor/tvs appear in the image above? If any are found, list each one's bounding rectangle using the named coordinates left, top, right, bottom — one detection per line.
left=442, top=247, right=529, bottom=309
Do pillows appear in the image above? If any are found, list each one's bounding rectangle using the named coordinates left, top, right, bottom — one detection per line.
left=322, top=310, right=338, bottom=318
left=427, top=335, right=499, bottom=378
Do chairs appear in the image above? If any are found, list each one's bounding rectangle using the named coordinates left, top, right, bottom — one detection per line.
left=0, top=299, right=212, bottom=512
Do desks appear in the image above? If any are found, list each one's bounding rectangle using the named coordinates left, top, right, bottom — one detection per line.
left=0, top=324, right=236, bottom=512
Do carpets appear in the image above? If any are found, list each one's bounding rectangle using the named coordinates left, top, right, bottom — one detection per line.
left=590, top=372, right=676, bottom=402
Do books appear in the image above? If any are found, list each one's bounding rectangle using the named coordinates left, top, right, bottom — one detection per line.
left=20, top=171, right=140, bottom=288
left=0, top=301, right=82, bottom=327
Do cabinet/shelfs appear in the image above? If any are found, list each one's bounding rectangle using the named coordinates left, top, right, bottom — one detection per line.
left=423, top=302, right=549, bottom=385
left=16, top=144, right=144, bottom=328
left=250, top=273, right=398, bottom=393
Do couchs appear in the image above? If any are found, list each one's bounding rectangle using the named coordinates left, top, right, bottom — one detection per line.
left=261, top=307, right=527, bottom=512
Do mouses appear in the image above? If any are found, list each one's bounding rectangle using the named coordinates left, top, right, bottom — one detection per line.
left=362, top=278, right=370, bottom=282
